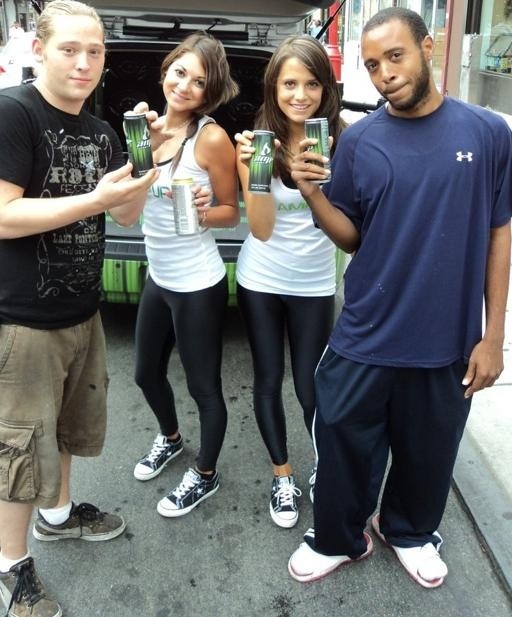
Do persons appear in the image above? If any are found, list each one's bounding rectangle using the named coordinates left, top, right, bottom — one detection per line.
left=233, top=32, right=352, bottom=531
left=284, top=5, right=511, bottom=590
left=307, top=16, right=326, bottom=45
left=130, top=28, right=244, bottom=518
left=0, top=0, right=183, bottom=615
left=8, top=20, right=25, bottom=38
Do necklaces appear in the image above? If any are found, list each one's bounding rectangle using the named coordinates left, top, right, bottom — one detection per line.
left=173, top=116, right=191, bottom=130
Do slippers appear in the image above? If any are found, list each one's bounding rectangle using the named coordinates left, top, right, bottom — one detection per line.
left=286, top=528, right=374, bottom=583
left=372, top=512, right=449, bottom=590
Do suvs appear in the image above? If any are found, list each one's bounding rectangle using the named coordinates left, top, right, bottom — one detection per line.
left=15, top=0, right=350, bottom=317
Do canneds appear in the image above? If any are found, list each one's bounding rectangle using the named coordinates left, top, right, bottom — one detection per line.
left=171, top=177, right=200, bottom=237
left=304, top=119, right=333, bottom=185
left=123, top=110, right=154, bottom=178
left=247, top=128, right=275, bottom=194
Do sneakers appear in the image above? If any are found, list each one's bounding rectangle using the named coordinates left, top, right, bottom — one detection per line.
left=268, top=470, right=300, bottom=529
left=156, top=463, right=222, bottom=521
left=307, top=466, right=318, bottom=506
left=133, top=429, right=185, bottom=482
left=0, top=546, right=64, bottom=617
left=31, top=501, right=128, bottom=546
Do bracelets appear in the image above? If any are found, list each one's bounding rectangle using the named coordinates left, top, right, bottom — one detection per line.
left=200, top=210, right=207, bottom=227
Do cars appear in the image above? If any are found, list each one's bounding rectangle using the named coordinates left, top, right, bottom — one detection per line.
left=1, top=32, right=44, bottom=88
